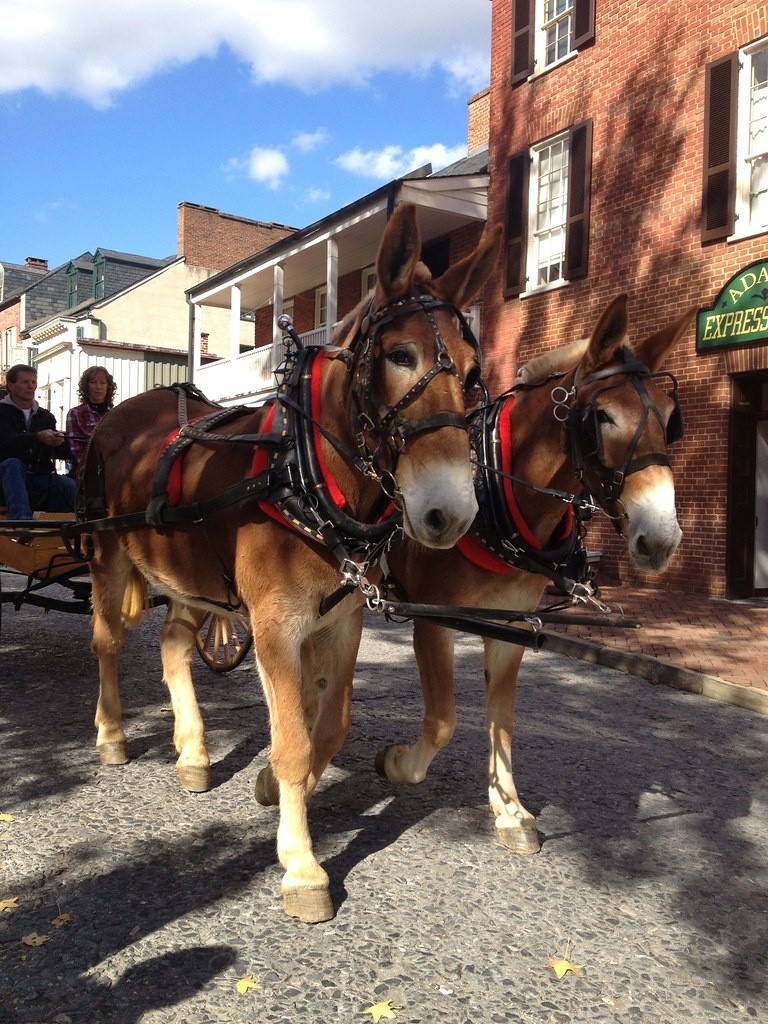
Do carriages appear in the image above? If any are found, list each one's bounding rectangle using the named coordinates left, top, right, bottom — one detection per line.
left=0, top=195, right=690, bottom=922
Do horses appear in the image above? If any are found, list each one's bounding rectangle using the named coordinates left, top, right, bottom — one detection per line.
left=292, top=294, right=701, bottom=840
left=81, top=201, right=509, bottom=921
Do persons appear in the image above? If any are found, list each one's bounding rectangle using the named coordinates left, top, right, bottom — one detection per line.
left=65, top=365, right=117, bottom=497
left=1, top=363, right=78, bottom=546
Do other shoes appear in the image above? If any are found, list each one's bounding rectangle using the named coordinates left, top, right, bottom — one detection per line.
left=5, top=518, right=35, bottom=548
